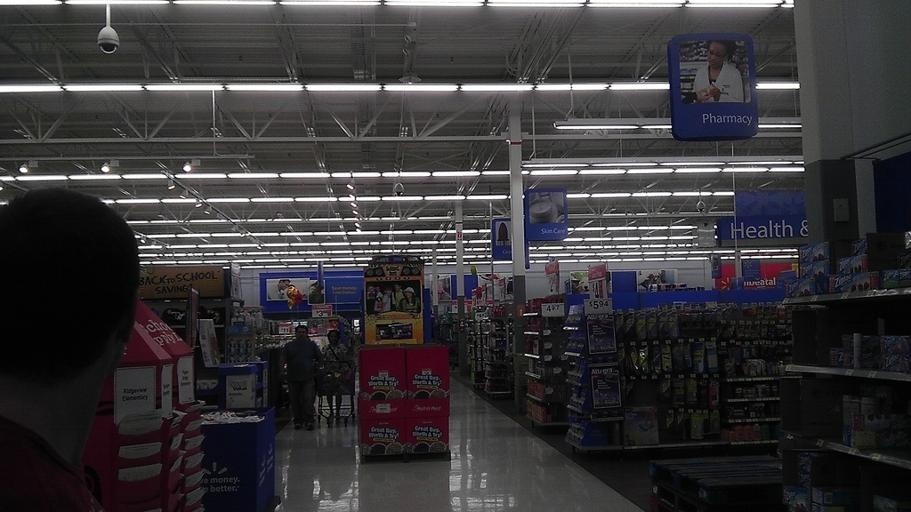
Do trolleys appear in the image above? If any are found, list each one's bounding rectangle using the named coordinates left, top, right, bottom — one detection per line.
left=311, top=358, right=360, bottom=427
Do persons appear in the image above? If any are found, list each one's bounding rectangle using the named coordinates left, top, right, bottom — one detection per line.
left=693, top=39, right=746, bottom=103
left=320, top=329, right=350, bottom=424
left=268, top=279, right=291, bottom=299
left=498, top=222, right=510, bottom=242
left=280, top=324, right=323, bottom=430
left=0, top=187, right=141, bottom=510
left=373, top=282, right=422, bottom=314
left=310, top=283, right=325, bottom=304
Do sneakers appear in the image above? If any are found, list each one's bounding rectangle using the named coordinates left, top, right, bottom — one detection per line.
left=305, top=422, right=315, bottom=432
left=294, top=422, right=304, bottom=429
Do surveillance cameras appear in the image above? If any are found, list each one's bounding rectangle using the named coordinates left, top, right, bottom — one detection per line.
left=394, top=183, right=404, bottom=196
left=97, top=26, right=120, bottom=54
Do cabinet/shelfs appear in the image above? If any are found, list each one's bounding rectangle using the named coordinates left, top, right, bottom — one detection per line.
left=781, top=289, right=910, bottom=512
left=141, top=299, right=244, bottom=409
left=457, top=295, right=792, bottom=453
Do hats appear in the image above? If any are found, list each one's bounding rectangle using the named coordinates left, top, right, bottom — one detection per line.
left=403, top=287, right=414, bottom=293
